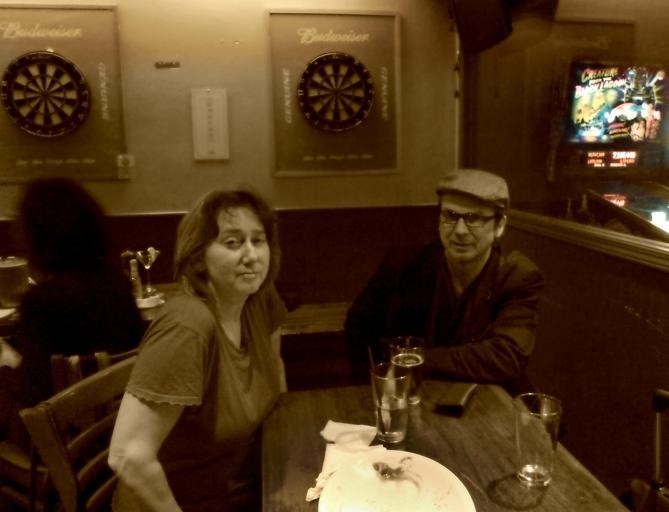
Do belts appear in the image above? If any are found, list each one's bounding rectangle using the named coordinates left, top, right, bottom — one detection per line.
left=134, top=250, right=161, bottom=299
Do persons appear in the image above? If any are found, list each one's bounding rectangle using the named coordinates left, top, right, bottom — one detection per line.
left=3, top=175, right=146, bottom=500
left=106, top=183, right=289, bottom=512
left=347, top=168, right=539, bottom=413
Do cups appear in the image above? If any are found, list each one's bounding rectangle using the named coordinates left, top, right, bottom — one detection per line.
left=439, top=207, right=497, bottom=227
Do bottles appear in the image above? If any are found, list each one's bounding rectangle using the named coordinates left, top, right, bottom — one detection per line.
left=436, top=169, right=511, bottom=210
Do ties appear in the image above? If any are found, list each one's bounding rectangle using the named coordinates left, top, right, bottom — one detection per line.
left=546, top=56, right=669, bottom=183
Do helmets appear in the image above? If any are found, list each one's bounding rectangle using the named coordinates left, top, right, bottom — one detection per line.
left=318, top=450, right=476, bottom=512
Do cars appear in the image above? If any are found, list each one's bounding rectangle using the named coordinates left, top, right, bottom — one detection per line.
left=371, top=335, right=426, bottom=444
left=512, top=392, right=564, bottom=489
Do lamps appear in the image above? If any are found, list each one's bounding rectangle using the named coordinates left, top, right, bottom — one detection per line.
left=0, top=347, right=139, bottom=512
